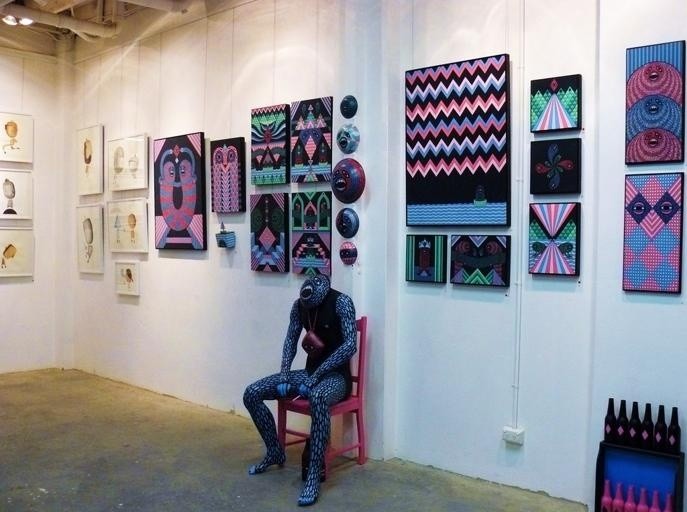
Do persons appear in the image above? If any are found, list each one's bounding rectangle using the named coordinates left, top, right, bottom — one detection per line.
left=242, top=272, right=358, bottom=505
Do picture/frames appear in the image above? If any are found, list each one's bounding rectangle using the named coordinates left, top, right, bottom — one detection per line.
left=71, top=122, right=104, bottom=197
left=76, top=204, right=104, bottom=275
left=0, top=112, right=34, bottom=162
left=0, top=226, right=34, bottom=278
left=106, top=134, right=149, bottom=193
left=104, top=198, right=149, bottom=254
left=113, top=260, right=140, bottom=296
left=0, top=168, right=33, bottom=220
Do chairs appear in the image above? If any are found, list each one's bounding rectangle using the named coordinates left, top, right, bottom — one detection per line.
left=270, top=314, right=369, bottom=479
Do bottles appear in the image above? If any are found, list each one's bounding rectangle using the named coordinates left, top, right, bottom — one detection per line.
left=599, top=479, right=672, bottom=511
left=602, top=396, right=680, bottom=456
